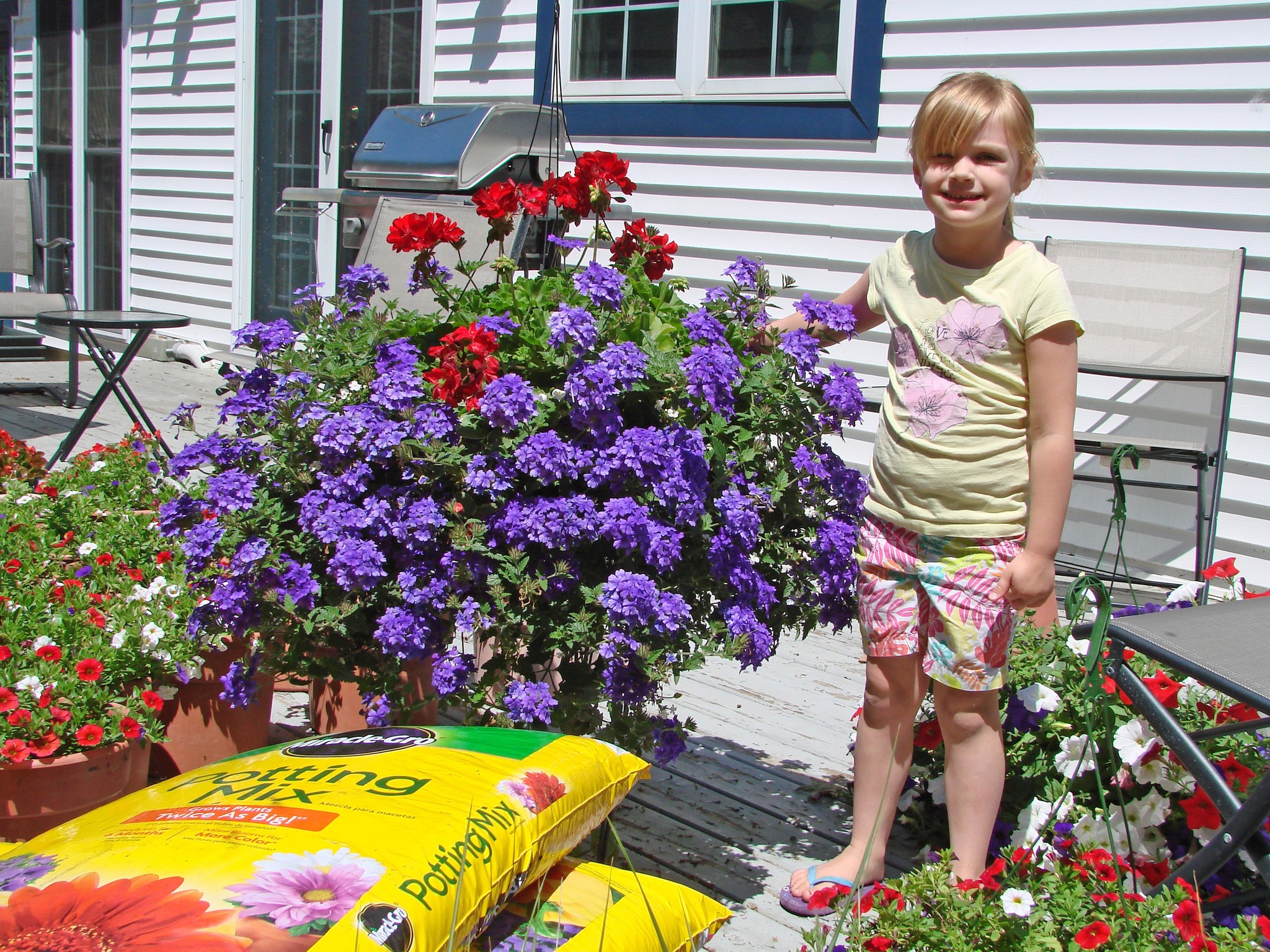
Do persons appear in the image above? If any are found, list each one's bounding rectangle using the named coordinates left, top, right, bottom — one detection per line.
left=747, top=74, right=1084, bottom=913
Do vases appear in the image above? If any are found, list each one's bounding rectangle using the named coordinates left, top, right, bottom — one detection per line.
left=1, top=695, right=134, bottom=844
left=304, top=642, right=440, bottom=734
left=472, top=623, right=604, bottom=710
left=265, top=615, right=343, bottom=692
left=120, top=627, right=285, bottom=780
left=92, top=675, right=152, bottom=797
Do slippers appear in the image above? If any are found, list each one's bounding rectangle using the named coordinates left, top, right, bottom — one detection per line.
left=780, top=865, right=887, bottom=916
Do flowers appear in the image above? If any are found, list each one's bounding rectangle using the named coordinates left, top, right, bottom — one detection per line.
left=0, top=145, right=1269, bottom=951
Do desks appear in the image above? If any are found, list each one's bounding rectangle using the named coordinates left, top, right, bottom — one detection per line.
left=37, top=310, right=192, bottom=475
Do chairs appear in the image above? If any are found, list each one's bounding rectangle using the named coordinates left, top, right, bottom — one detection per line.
left=1045, top=236, right=1246, bottom=611
left=1071, top=596, right=1270, bottom=921
left=0, top=171, right=78, bottom=408
